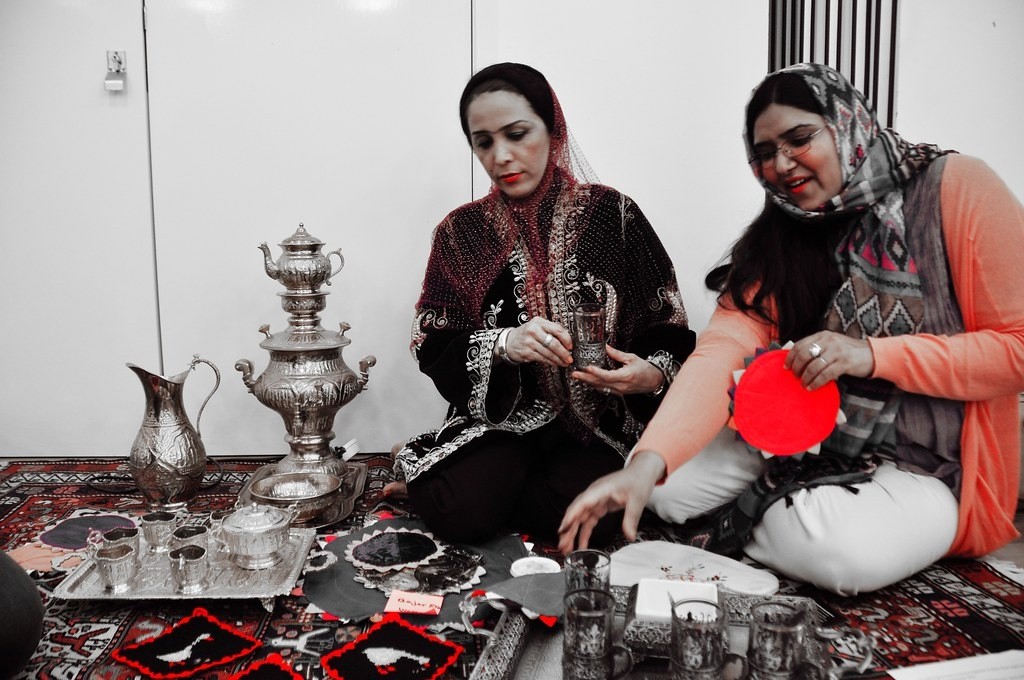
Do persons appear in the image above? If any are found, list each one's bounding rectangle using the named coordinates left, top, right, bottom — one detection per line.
left=377, top=62, right=696, bottom=547
left=559, top=63, right=1024, bottom=597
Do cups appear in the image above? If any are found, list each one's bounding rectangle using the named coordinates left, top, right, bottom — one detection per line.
left=168, top=545, right=208, bottom=594
left=87, top=527, right=140, bottom=558
left=210, top=509, right=234, bottom=549
left=210, top=503, right=299, bottom=569
left=141, top=512, right=175, bottom=553
left=571, top=303, right=610, bottom=370
left=86, top=543, right=136, bottom=589
left=747, top=603, right=807, bottom=680
left=564, top=549, right=610, bottom=593
left=671, top=599, right=747, bottom=680
left=171, top=525, right=211, bottom=570
left=563, top=589, right=633, bottom=680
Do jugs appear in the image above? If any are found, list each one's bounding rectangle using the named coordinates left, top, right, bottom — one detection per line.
left=125, top=355, right=220, bottom=512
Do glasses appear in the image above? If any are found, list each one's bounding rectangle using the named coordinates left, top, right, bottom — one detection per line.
left=749, top=126, right=826, bottom=171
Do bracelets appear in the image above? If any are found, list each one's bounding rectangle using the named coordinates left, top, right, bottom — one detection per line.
left=499, top=327, right=520, bottom=367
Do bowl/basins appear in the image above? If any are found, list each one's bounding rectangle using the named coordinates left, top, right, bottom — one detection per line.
left=248, top=473, right=342, bottom=525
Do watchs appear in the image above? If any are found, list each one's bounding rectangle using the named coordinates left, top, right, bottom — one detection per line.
left=653, top=371, right=666, bottom=395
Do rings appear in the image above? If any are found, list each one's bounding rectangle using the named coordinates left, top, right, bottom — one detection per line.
left=809, top=343, right=821, bottom=357
left=543, top=333, right=553, bottom=347
left=602, top=388, right=611, bottom=395
left=818, top=356, right=828, bottom=367
